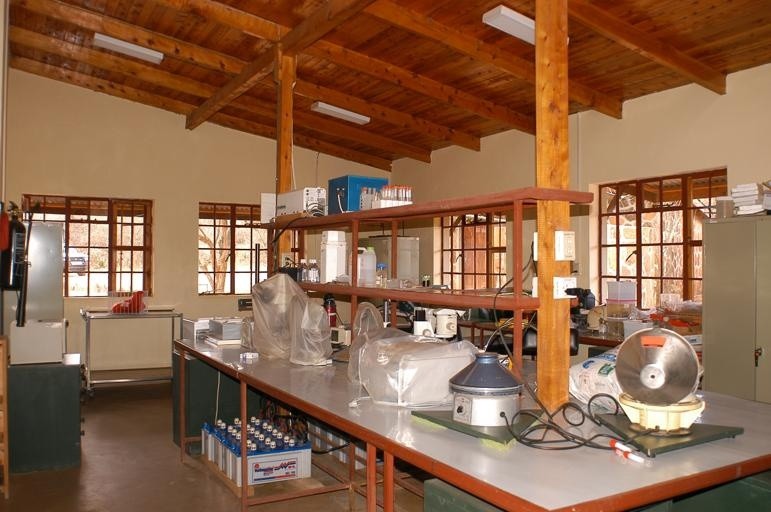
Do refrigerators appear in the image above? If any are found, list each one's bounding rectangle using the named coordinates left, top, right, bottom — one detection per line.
left=1, top=219, right=64, bottom=364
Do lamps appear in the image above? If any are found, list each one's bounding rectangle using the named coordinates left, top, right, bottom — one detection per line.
left=311, top=101, right=370, bottom=126
left=482, top=5, right=569, bottom=47
left=93, top=32, right=164, bottom=66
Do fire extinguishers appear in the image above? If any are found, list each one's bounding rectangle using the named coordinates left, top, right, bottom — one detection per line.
left=0, top=200, right=26, bottom=290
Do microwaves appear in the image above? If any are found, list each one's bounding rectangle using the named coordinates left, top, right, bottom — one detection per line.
left=9, top=317, right=69, bottom=365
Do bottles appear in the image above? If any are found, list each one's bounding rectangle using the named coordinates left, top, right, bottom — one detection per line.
left=375, top=263, right=388, bottom=289
left=214, top=416, right=299, bottom=455
left=298, top=259, right=320, bottom=283
left=359, top=246, right=377, bottom=288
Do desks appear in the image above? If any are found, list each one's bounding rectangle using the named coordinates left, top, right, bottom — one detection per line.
left=576, top=332, right=620, bottom=357
left=578, top=332, right=622, bottom=347
left=174, top=335, right=771, bottom=512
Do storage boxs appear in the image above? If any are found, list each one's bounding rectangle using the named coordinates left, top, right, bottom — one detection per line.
left=606, top=280, right=637, bottom=338
left=307, top=420, right=384, bottom=471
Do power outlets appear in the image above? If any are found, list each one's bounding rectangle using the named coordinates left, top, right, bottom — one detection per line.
left=555, top=231, right=575, bottom=261
left=554, top=277, right=578, bottom=299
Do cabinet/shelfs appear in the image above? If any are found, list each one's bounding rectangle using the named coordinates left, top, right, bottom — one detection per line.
left=262, top=187, right=593, bottom=409
left=701, top=215, right=771, bottom=405
